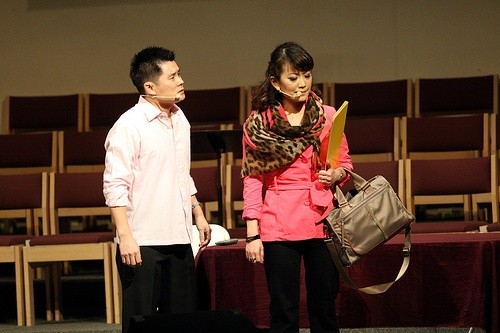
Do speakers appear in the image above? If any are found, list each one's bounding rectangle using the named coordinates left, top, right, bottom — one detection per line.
left=127, top=307, right=260, bottom=333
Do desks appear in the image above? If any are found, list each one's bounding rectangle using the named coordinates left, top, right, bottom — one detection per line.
left=195, top=232, right=500, bottom=332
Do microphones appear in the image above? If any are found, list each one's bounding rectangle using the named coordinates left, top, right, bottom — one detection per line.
left=142, top=93, right=181, bottom=100
left=276, top=86, right=301, bottom=98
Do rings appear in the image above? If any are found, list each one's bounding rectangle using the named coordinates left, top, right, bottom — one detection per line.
left=250, top=258, right=256, bottom=262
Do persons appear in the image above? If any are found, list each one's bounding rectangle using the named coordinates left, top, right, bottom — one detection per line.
left=243, top=42, right=353, bottom=333
left=102, top=48, right=211, bottom=333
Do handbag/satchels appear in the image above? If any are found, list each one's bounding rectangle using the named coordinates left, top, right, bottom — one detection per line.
left=322, top=168, right=415, bottom=295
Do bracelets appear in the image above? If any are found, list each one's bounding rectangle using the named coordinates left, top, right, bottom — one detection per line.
left=338, top=169, right=343, bottom=181
left=192, top=202, right=202, bottom=209
left=246, top=235, right=260, bottom=242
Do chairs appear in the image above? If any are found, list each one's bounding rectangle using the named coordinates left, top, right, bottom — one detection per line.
left=0, top=75, right=500, bottom=324
